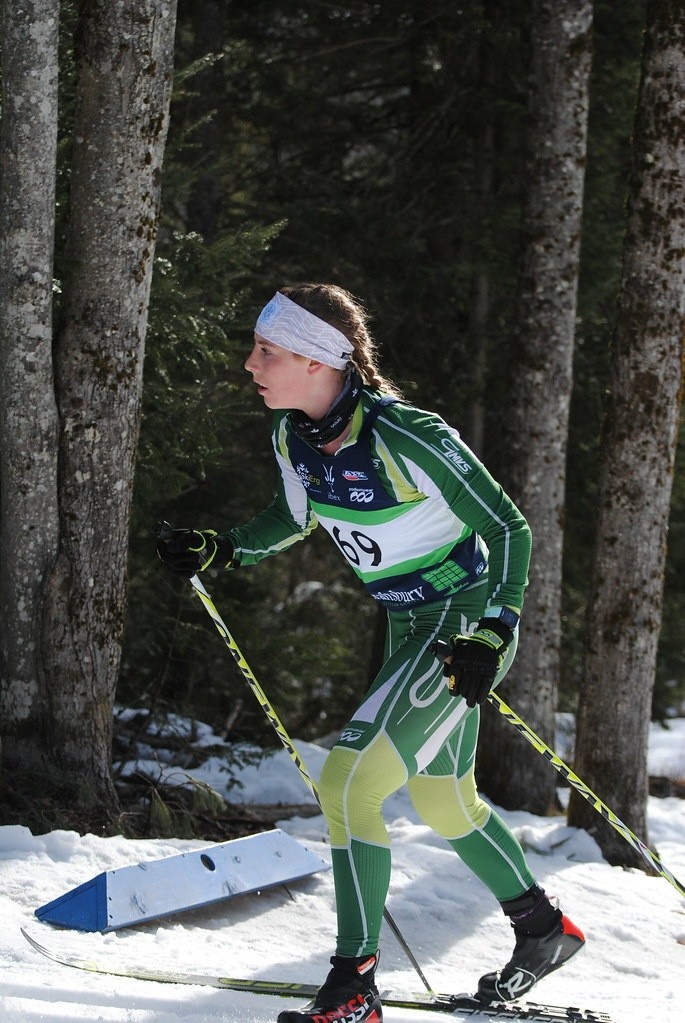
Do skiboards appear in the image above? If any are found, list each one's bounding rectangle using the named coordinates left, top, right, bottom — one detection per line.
left=19, top=926, right=616, bottom=1023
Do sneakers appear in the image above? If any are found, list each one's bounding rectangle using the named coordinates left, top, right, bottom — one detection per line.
left=479, top=896, right=585, bottom=1002
left=276, top=949, right=383, bottom=1023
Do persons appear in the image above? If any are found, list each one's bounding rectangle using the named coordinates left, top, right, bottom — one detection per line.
left=155, top=283, right=585, bottom=1023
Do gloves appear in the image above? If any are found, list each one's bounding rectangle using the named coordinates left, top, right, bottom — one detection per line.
left=157, top=528, right=235, bottom=578
left=443, top=617, right=514, bottom=708
